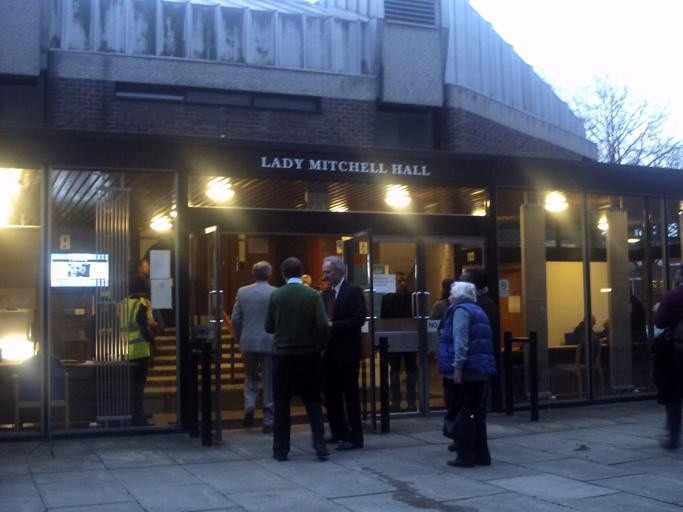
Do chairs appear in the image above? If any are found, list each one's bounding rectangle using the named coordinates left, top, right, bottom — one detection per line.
left=13, top=355, right=70, bottom=432
left=554, top=340, right=605, bottom=399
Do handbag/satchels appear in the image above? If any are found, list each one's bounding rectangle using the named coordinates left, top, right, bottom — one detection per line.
left=443, top=407, right=483, bottom=440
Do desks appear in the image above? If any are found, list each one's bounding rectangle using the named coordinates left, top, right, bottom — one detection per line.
left=502, top=341, right=608, bottom=354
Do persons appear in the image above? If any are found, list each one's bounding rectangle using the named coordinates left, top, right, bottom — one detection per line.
left=573, top=314, right=596, bottom=335
left=380, top=271, right=421, bottom=412
left=135, top=255, right=165, bottom=370
left=430, top=279, right=455, bottom=332
left=651, top=262, right=683, bottom=452
left=629, top=294, right=648, bottom=344
left=597, top=319, right=609, bottom=339
left=444, top=264, right=500, bottom=451
left=263, top=256, right=329, bottom=462
left=437, top=280, right=496, bottom=468
left=84, top=296, right=121, bottom=358
left=301, top=274, right=313, bottom=287
left=230, top=259, right=280, bottom=434
left=117, top=273, right=155, bottom=427
left=313, top=254, right=366, bottom=452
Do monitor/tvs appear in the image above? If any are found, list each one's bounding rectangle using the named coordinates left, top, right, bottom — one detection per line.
left=50, top=251, right=110, bottom=289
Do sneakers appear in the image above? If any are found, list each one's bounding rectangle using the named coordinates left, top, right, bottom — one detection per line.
left=133, top=418, right=155, bottom=426
left=272, top=454, right=287, bottom=460
left=448, top=444, right=491, bottom=466
left=316, top=436, right=359, bottom=457
left=243, top=406, right=255, bottom=427
